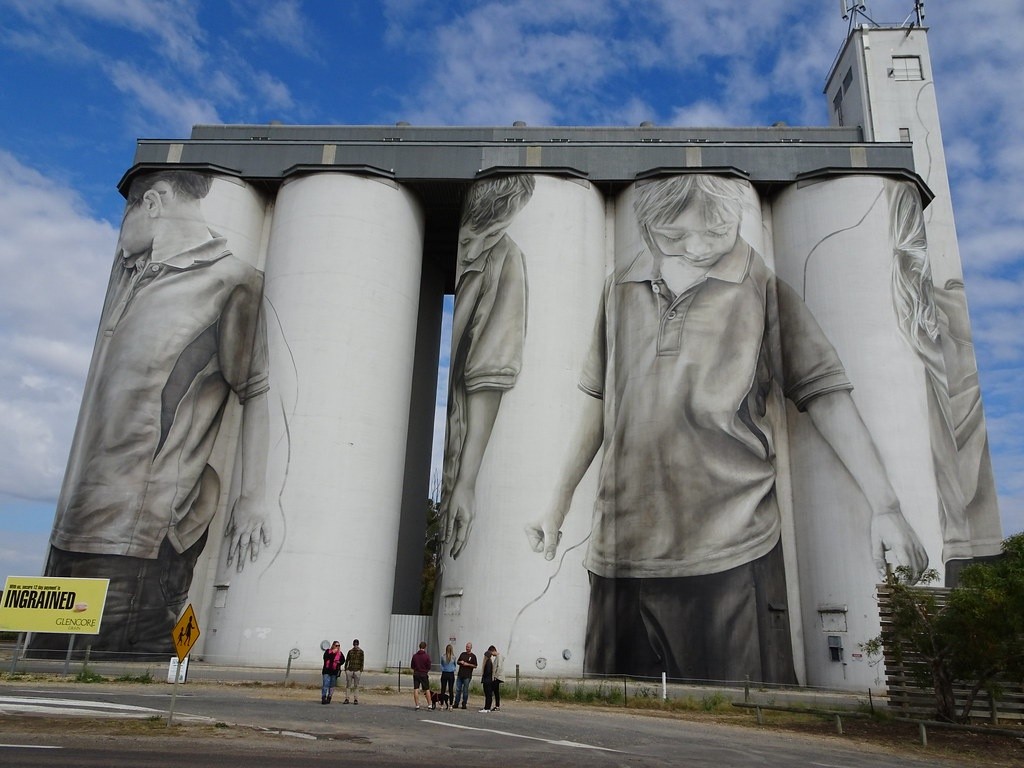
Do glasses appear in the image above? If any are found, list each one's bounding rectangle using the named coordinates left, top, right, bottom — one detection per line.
left=334, top=645, right=340, bottom=647
left=354, top=644, right=358, bottom=646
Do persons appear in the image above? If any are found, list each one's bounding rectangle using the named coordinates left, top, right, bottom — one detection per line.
left=885, top=183, right=1002, bottom=591
left=524, top=173, right=930, bottom=686
left=343, top=639, right=364, bottom=705
left=321, top=641, right=345, bottom=704
left=452, top=642, right=477, bottom=709
left=26, top=160, right=273, bottom=659
left=440, top=644, right=457, bottom=712
left=443, top=173, right=536, bottom=560
left=411, top=642, right=432, bottom=711
left=479, top=646, right=505, bottom=713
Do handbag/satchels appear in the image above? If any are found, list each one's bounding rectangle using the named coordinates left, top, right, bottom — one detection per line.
left=337, top=669, right=341, bottom=677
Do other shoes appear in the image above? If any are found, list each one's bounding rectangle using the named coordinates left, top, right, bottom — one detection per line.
left=344, top=698, right=349, bottom=704
left=478, top=708, right=491, bottom=713
left=354, top=699, right=358, bottom=704
left=462, top=706, right=466, bottom=709
left=415, top=707, right=420, bottom=712
left=449, top=706, right=453, bottom=711
left=321, top=696, right=331, bottom=704
left=428, top=706, right=434, bottom=712
left=491, top=707, right=500, bottom=711
left=440, top=706, right=444, bottom=712
left=453, top=705, right=458, bottom=708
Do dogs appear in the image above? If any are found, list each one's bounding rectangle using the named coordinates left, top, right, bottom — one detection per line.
left=423, top=690, right=450, bottom=711
